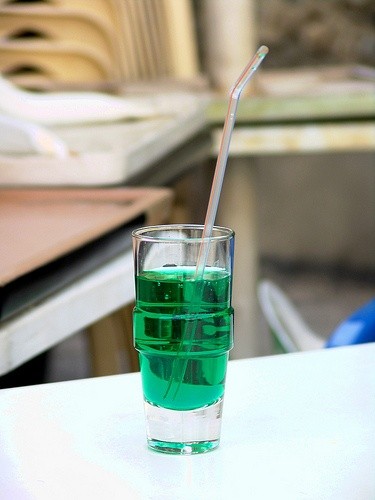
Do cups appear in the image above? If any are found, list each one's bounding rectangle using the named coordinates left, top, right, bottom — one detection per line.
left=131, top=224, right=235, bottom=454
